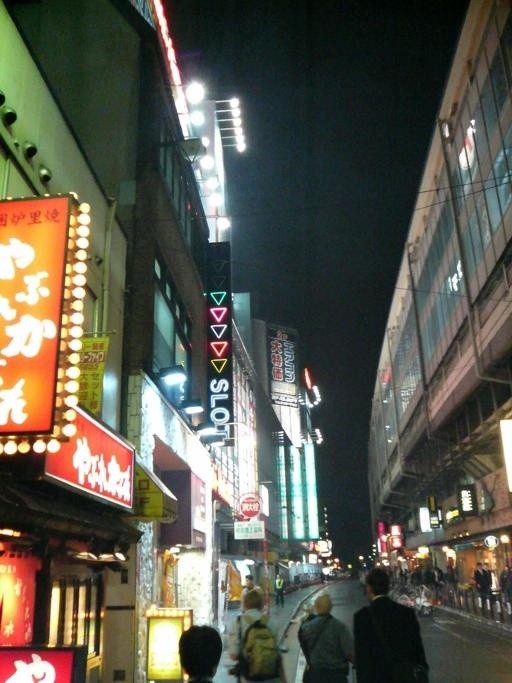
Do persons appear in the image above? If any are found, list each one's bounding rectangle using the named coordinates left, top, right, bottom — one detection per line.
left=359, top=562, right=512, bottom=624
left=229, top=607, right=287, bottom=683
left=179, top=624, right=222, bottom=683
left=241, top=574, right=265, bottom=613
left=274, top=574, right=285, bottom=608
left=298, top=595, right=355, bottom=683
left=352, top=568, right=429, bottom=683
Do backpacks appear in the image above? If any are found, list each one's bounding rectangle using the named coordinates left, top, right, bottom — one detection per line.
left=240, top=613, right=282, bottom=681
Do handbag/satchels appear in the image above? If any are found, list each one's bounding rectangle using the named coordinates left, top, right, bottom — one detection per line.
left=399, top=662, right=429, bottom=683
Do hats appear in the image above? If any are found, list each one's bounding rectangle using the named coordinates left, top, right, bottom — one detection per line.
left=315, top=595, right=331, bottom=611
left=245, top=591, right=262, bottom=609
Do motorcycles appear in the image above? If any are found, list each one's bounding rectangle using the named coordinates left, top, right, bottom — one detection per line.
left=392, top=584, right=433, bottom=616
